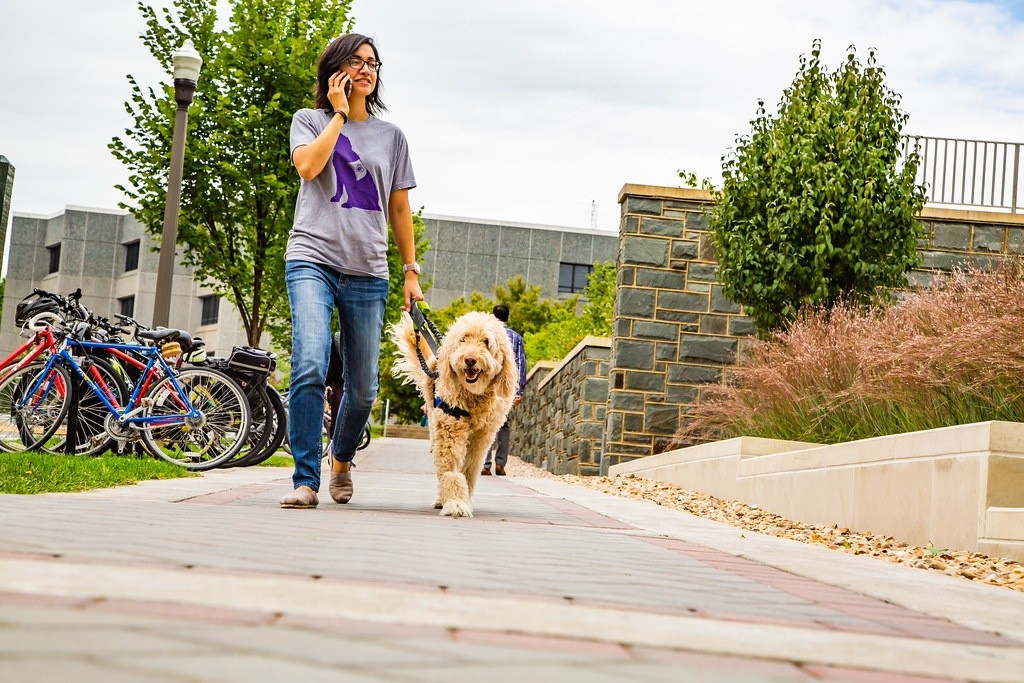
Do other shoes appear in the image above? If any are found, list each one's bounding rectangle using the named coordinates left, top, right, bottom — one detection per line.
left=496, top=465, right=506, bottom=475
left=328, top=446, right=353, bottom=503
left=481, top=468, right=491, bottom=475
left=280, top=488, right=319, bottom=508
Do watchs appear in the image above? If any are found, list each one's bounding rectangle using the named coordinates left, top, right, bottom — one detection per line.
left=402, top=262, right=420, bottom=276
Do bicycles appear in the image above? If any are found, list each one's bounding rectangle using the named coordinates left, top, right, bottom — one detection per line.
left=0, top=288, right=374, bottom=471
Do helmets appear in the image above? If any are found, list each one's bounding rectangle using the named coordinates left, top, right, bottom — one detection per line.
left=14, top=297, right=59, bottom=329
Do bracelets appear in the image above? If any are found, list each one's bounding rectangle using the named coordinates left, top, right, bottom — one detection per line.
left=333, top=110, right=348, bottom=124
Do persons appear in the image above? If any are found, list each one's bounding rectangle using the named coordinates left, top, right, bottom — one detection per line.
left=280, top=30, right=424, bottom=512
left=480, top=304, right=527, bottom=475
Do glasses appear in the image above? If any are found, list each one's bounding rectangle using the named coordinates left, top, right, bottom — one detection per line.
left=348, top=57, right=382, bottom=71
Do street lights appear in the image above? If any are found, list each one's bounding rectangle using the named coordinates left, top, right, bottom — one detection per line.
left=150, top=37, right=202, bottom=332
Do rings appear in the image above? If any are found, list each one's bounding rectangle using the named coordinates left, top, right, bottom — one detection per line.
left=328, top=83, right=333, bottom=85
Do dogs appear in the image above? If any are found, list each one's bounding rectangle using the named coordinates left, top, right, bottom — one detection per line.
left=383, top=309, right=520, bottom=519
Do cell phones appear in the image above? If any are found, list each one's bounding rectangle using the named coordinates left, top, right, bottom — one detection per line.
left=337, top=73, right=352, bottom=98
